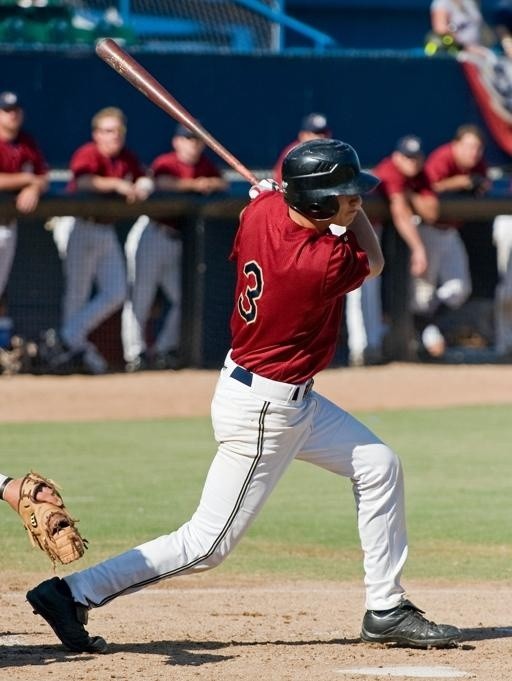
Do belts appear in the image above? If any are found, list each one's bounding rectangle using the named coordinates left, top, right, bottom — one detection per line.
left=232, top=367, right=313, bottom=399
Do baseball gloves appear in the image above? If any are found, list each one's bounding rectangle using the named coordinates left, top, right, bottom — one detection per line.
left=18, top=471, right=86, bottom=566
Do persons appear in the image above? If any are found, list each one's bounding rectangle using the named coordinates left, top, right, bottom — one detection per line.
left=428, top=0, right=485, bottom=51
left=25, top=139, right=461, bottom=653
left=408, top=124, right=487, bottom=364
left=274, top=111, right=333, bottom=190
left=51, top=107, right=157, bottom=375
left=1, top=92, right=52, bottom=299
left=0, top=471, right=86, bottom=566
left=120, top=122, right=229, bottom=373
left=360, top=135, right=441, bottom=366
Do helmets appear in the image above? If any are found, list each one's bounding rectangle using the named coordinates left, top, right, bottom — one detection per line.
left=282, top=138, right=381, bottom=221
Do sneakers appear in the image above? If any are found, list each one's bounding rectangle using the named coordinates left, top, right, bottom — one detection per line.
left=26, top=577, right=106, bottom=654
left=360, top=599, right=462, bottom=647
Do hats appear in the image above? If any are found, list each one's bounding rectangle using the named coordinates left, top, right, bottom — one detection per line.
left=0, top=92, right=21, bottom=110
left=300, top=113, right=333, bottom=133
left=397, top=135, right=423, bottom=156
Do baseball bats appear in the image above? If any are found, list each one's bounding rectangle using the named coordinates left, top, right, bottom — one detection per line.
left=96, top=37, right=259, bottom=186
